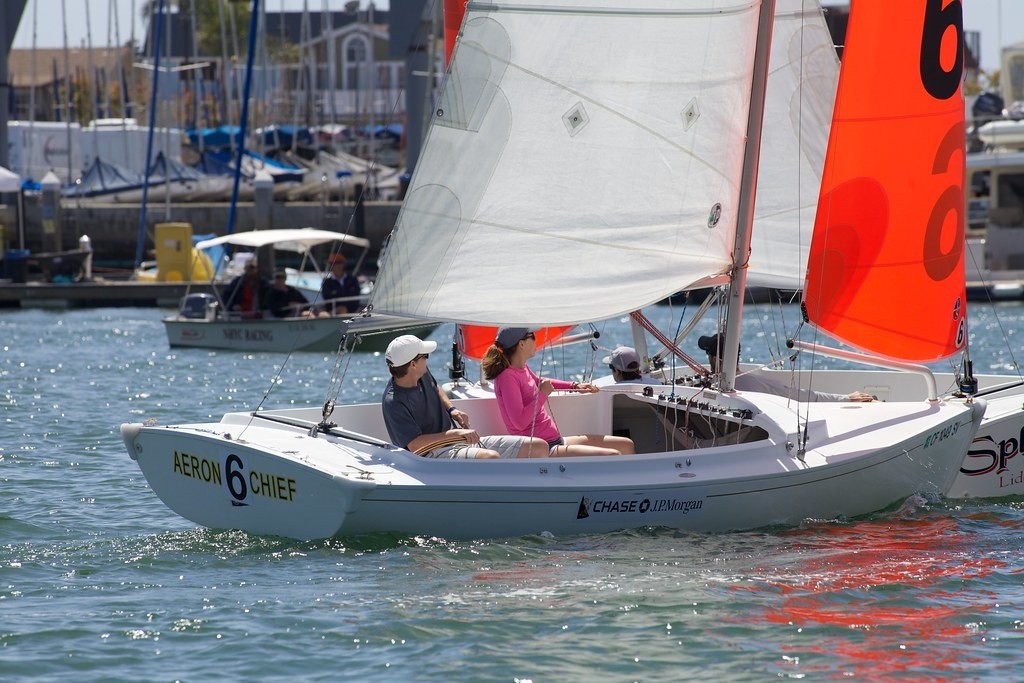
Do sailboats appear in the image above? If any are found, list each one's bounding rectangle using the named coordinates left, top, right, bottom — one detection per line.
left=119, top=1, right=1024, bottom=541
left=60, top=0, right=400, bottom=204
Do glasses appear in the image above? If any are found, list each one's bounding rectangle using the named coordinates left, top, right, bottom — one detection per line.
left=520, top=332, right=536, bottom=340
left=414, top=353, right=429, bottom=361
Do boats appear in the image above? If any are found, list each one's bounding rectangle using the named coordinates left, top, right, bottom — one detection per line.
left=160, top=228, right=444, bottom=354
left=278, top=272, right=374, bottom=311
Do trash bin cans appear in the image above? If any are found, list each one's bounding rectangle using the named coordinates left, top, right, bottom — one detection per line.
left=4, top=249, right=31, bottom=284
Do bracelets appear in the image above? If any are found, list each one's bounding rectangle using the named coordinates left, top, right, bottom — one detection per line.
left=448, top=406, right=457, bottom=414
left=574, top=382, right=578, bottom=392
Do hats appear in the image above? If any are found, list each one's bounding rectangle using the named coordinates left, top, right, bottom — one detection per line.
left=698, top=332, right=740, bottom=358
left=245, top=259, right=257, bottom=267
left=328, top=253, right=344, bottom=264
left=602, top=346, right=641, bottom=371
left=385, top=334, right=437, bottom=367
left=273, top=266, right=286, bottom=277
left=496, top=327, right=541, bottom=348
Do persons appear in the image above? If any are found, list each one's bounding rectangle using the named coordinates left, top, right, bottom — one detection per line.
left=222, top=259, right=267, bottom=312
left=381, top=335, right=550, bottom=460
left=603, top=346, right=662, bottom=385
left=263, top=270, right=317, bottom=317
left=481, top=326, right=634, bottom=458
left=322, top=254, right=361, bottom=315
left=698, top=332, right=873, bottom=402
